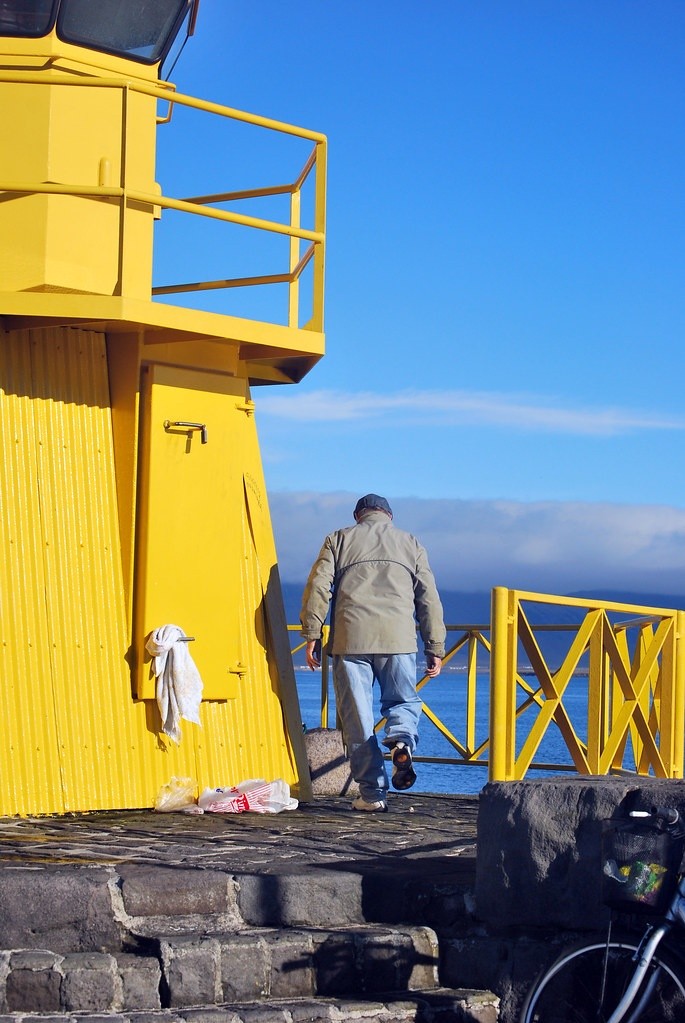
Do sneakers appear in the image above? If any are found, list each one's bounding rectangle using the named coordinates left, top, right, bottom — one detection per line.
left=391, top=742, right=417, bottom=791
left=351, top=796, right=388, bottom=812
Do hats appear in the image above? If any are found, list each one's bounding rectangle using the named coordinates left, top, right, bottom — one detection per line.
left=356, top=494, right=392, bottom=514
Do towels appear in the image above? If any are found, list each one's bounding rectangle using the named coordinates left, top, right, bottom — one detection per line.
left=144, top=624, right=206, bottom=750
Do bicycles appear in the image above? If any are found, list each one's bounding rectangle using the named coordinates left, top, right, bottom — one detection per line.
left=521, top=804, right=685, bottom=1023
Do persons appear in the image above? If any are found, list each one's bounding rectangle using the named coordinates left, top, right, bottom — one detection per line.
left=299, top=494, right=447, bottom=812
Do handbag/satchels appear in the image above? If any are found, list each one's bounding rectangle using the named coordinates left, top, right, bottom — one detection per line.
left=155, top=776, right=196, bottom=810
left=197, top=777, right=298, bottom=815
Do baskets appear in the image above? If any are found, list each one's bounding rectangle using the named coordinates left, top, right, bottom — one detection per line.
left=599, top=815, right=681, bottom=918
